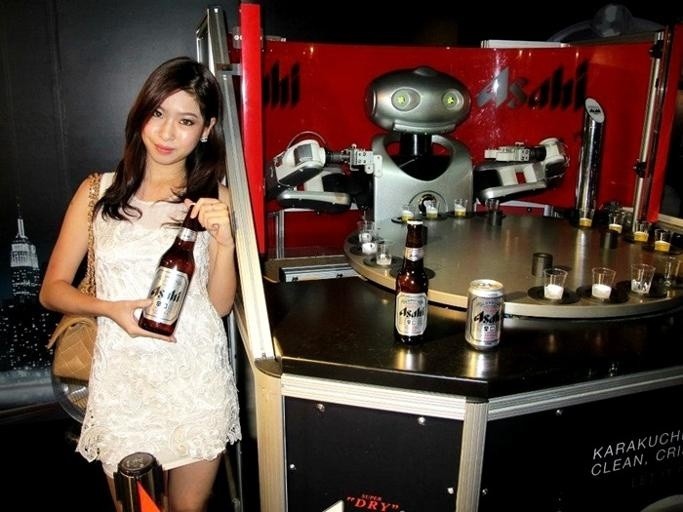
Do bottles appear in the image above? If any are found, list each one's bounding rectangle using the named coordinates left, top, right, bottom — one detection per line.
left=395, top=220, right=428, bottom=346
left=138, top=205, right=199, bottom=337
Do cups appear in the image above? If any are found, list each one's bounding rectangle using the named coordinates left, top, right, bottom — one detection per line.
left=402, top=203, right=415, bottom=224
left=633, top=217, right=652, bottom=242
left=454, top=197, right=468, bottom=218
left=664, top=255, right=682, bottom=283
left=578, top=207, right=595, bottom=229
left=590, top=266, right=618, bottom=302
left=653, top=228, right=673, bottom=254
left=542, top=266, right=569, bottom=302
left=356, top=220, right=375, bottom=243
left=607, top=211, right=626, bottom=236
left=375, top=240, right=396, bottom=267
left=629, top=262, right=657, bottom=296
left=360, top=231, right=380, bottom=255
left=423, top=200, right=441, bottom=220
left=486, top=198, right=501, bottom=211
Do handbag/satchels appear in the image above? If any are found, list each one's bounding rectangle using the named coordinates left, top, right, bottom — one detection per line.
left=46, top=170, right=100, bottom=380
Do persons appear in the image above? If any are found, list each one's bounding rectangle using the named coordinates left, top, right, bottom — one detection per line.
left=39, top=54, right=238, bottom=512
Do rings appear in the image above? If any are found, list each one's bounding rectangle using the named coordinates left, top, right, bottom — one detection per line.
left=209, top=203, right=217, bottom=209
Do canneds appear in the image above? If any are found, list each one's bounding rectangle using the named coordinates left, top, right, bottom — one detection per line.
left=465, top=278, right=504, bottom=350
